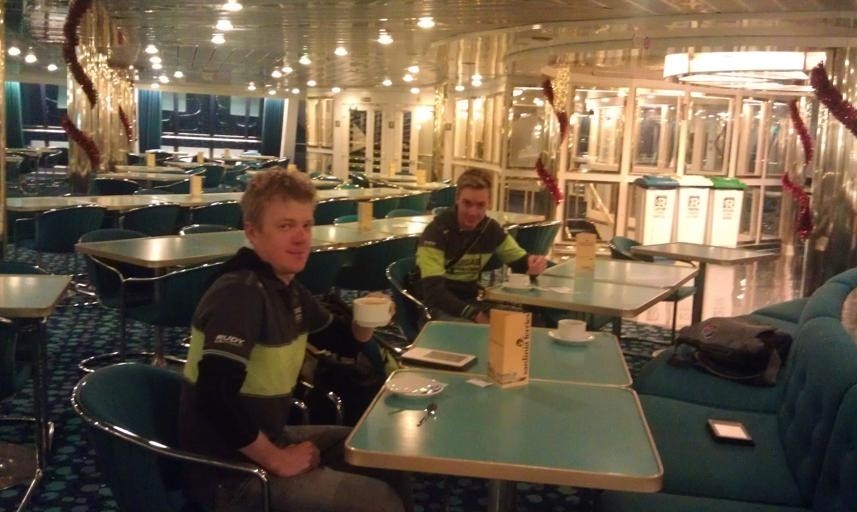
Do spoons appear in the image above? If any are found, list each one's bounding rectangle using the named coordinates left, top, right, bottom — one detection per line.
left=417, top=403, right=437, bottom=427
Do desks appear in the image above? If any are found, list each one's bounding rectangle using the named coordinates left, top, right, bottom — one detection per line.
left=345, top=367, right=662, bottom=507
left=390, top=317, right=635, bottom=387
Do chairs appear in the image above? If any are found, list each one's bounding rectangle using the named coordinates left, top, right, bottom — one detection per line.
left=77, top=348, right=345, bottom=425
left=0, top=264, right=74, bottom=512
left=388, top=218, right=780, bottom=357
left=74, top=367, right=272, bottom=509
left=1, top=142, right=561, bottom=365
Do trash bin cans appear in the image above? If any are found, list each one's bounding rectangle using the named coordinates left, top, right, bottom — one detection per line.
left=634, top=174, right=747, bottom=260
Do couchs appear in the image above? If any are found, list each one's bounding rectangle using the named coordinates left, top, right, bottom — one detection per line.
left=603, top=263, right=856, bottom=512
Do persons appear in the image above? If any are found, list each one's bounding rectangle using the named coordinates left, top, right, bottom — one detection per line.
left=177, top=168, right=415, bottom=511
left=407, top=166, right=548, bottom=324
left=801, top=170, right=857, bottom=279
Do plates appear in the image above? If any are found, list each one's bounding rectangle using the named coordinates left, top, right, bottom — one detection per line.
left=386, top=374, right=443, bottom=399
left=548, top=328, right=594, bottom=345
left=503, top=282, right=536, bottom=293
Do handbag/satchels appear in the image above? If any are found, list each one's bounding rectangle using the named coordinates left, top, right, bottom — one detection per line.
left=299, top=287, right=407, bottom=410
left=669, top=316, right=793, bottom=386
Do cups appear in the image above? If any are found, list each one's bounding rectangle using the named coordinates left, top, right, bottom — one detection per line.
left=353, top=297, right=390, bottom=328
left=556, top=320, right=587, bottom=341
left=509, top=274, right=530, bottom=288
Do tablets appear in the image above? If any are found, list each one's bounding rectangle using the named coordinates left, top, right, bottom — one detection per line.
left=401, top=348, right=476, bottom=370
left=707, top=418, right=752, bottom=442
